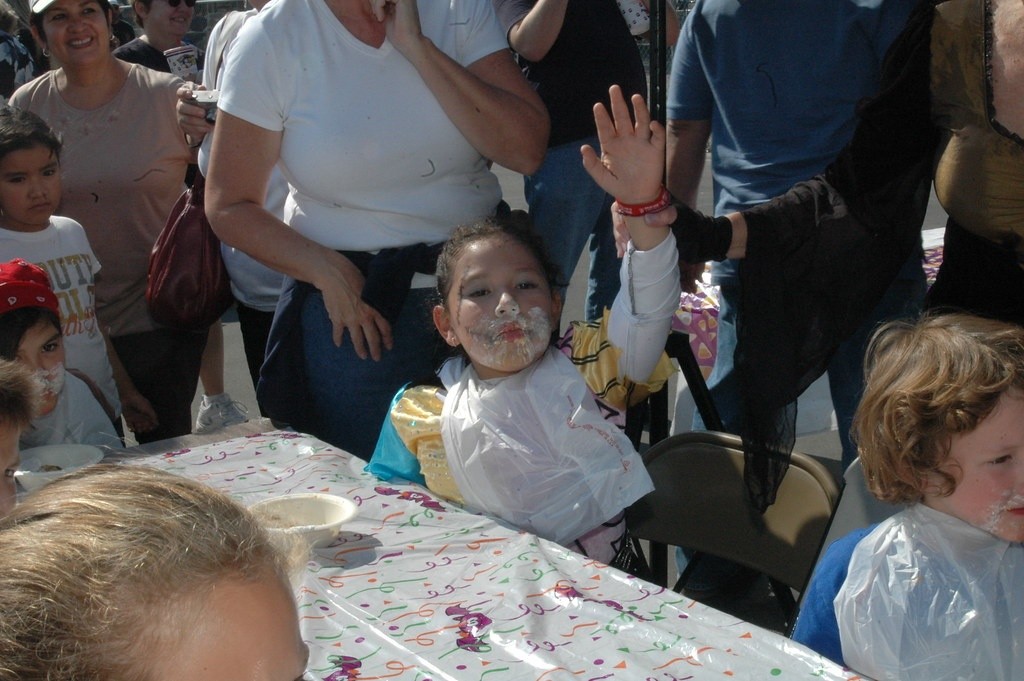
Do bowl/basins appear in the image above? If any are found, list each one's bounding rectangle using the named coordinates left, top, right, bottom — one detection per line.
left=247, top=492, right=358, bottom=549
left=14, top=444, right=104, bottom=491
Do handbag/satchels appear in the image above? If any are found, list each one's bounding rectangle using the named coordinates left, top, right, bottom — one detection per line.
left=144, top=181, right=232, bottom=325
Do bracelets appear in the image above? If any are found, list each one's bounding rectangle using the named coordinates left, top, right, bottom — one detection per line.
left=615, top=182, right=671, bottom=218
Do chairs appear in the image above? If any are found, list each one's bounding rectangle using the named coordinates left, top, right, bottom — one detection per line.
left=625, top=430, right=840, bottom=640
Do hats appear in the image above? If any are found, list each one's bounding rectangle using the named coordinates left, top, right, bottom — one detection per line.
left=108, top=0, right=119, bottom=5
left=0, top=257, right=59, bottom=313
left=28, top=0, right=55, bottom=13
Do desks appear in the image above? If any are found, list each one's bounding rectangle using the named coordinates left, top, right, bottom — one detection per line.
left=0, top=417, right=878, bottom=681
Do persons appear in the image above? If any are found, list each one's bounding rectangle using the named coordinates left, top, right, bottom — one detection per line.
left=0, top=0, right=1024, bottom=640
left=790, top=308, right=1024, bottom=681
left=367, top=83, right=683, bottom=589
left=0, top=257, right=128, bottom=475
left=203, top=0, right=553, bottom=464
left=0, top=97, right=165, bottom=485
left=0, top=431, right=312, bottom=681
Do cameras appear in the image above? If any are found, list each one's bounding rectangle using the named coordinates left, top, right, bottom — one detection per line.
left=190, top=90, right=223, bottom=123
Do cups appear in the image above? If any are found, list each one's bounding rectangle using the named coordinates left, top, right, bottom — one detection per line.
left=163, top=45, right=198, bottom=86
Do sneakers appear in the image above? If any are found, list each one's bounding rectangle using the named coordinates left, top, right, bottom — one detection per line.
left=195, top=394, right=250, bottom=431
